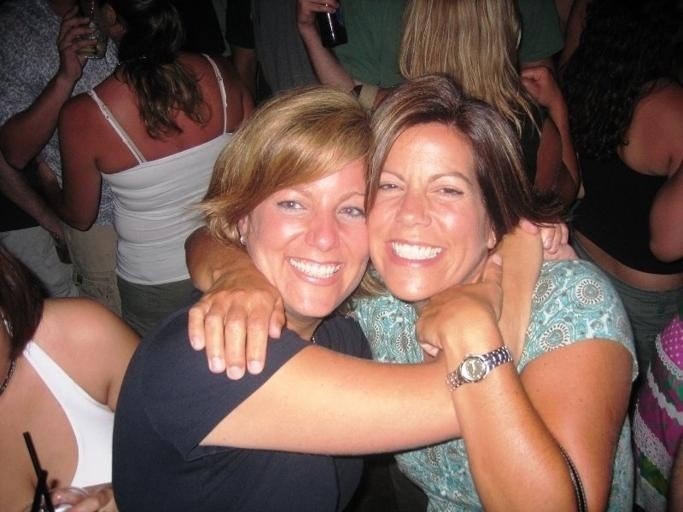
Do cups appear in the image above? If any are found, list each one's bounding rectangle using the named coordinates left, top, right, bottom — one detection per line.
left=75, top=16, right=108, bottom=60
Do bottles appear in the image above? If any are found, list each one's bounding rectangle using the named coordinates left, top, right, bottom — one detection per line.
left=317, top=0, right=348, bottom=48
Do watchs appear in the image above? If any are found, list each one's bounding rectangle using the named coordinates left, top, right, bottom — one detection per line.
left=446, top=346, right=514, bottom=391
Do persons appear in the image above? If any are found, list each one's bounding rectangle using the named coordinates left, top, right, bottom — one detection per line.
left=1, top=0, right=317, bottom=512
left=296, top=1, right=681, bottom=370
left=184, top=75, right=639, bottom=512
left=630, top=310, right=681, bottom=512
left=112, top=86, right=577, bottom=511
